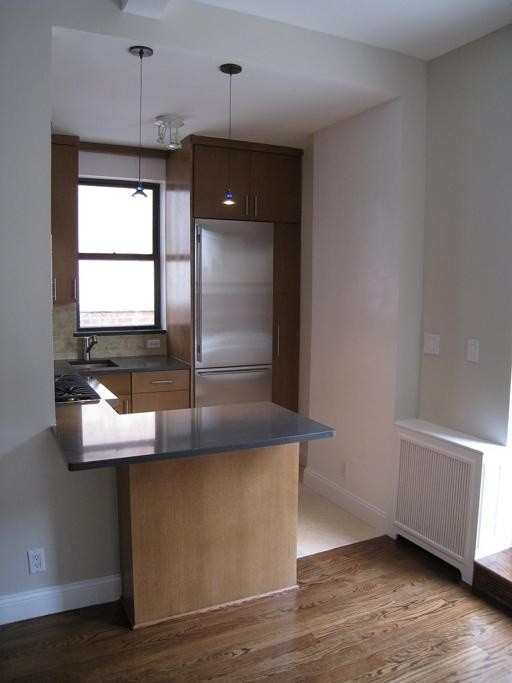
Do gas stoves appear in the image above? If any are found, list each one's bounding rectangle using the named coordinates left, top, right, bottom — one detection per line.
left=54, top=373, right=101, bottom=405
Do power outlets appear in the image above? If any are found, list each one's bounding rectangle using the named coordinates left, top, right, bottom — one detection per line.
left=27, top=545, right=51, bottom=580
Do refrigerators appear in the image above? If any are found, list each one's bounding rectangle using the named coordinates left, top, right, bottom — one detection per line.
left=193, top=217, right=274, bottom=409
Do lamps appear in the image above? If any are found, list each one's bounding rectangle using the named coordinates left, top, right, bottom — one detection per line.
left=219, top=63, right=241, bottom=209
left=130, top=45, right=153, bottom=203
left=156, top=112, right=184, bottom=152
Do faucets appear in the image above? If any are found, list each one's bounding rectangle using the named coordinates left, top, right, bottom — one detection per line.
left=82, top=336, right=97, bottom=359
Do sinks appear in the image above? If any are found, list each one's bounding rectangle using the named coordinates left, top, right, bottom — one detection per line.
left=66, top=360, right=118, bottom=370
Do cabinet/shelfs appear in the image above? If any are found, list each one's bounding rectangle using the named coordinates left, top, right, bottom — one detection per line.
left=54, top=129, right=300, bottom=632
